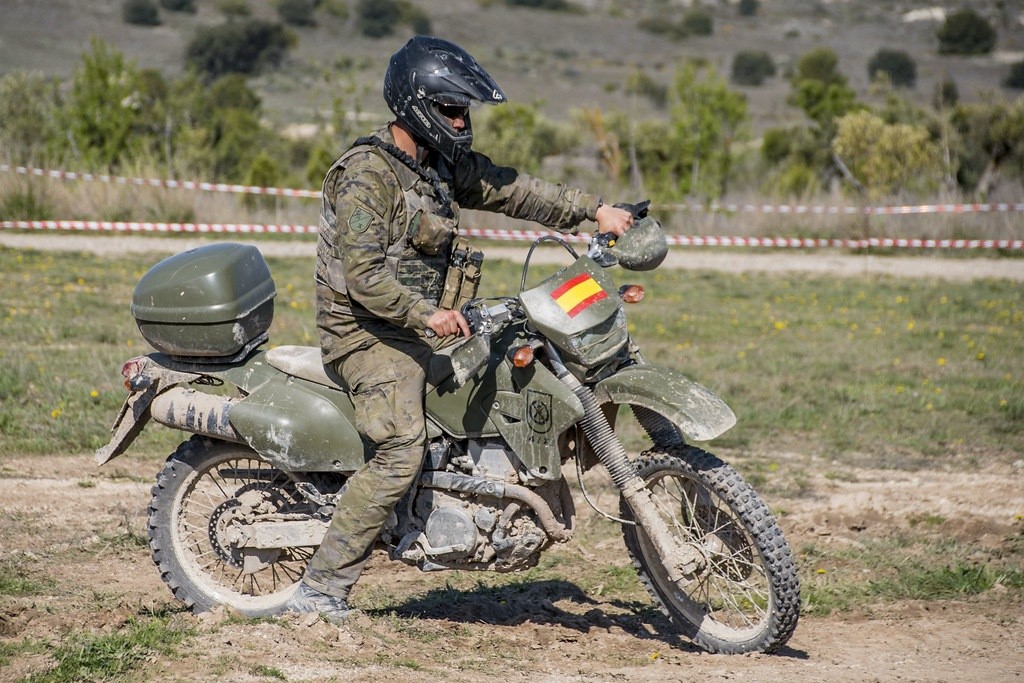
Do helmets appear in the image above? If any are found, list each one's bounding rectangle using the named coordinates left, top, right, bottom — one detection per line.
left=383, top=36, right=506, bottom=162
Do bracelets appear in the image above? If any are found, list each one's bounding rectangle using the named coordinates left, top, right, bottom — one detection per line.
left=599, top=200, right=603, bottom=206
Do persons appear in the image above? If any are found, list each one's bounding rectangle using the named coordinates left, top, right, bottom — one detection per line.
left=282, top=35, right=633, bottom=628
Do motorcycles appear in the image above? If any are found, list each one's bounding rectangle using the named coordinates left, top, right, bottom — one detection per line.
left=96, top=200, right=799, bottom=654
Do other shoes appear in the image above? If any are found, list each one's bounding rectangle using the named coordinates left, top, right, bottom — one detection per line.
left=287, top=583, right=354, bottom=625
